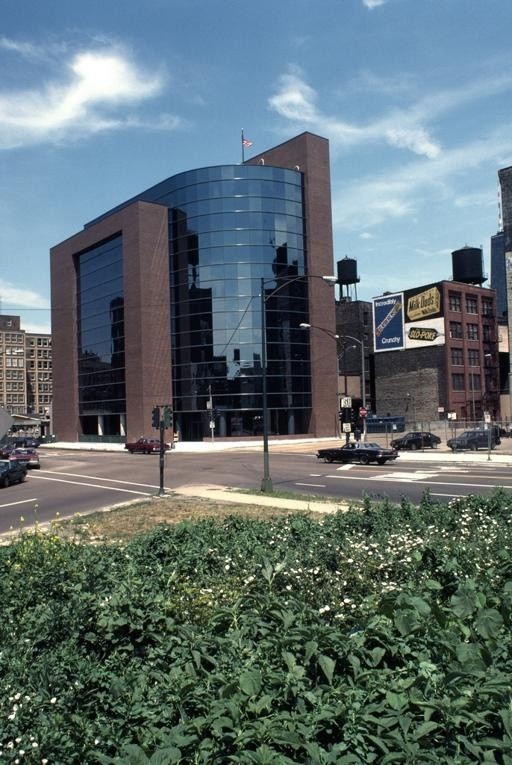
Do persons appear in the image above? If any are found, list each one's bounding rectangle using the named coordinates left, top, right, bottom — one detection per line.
left=353, top=427, right=361, bottom=441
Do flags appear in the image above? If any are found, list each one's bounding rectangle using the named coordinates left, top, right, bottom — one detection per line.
left=242, top=135, right=253, bottom=147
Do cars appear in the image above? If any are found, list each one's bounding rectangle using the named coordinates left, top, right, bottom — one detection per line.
left=390, top=432, right=441, bottom=450
left=316, top=442, right=400, bottom=465
left=446, top=430, right=502, bottom=450
left=0, top=437, right=40, bottom=489
left=125, top=439, right=171, bottom=454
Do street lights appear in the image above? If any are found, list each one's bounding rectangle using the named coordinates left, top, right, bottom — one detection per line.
left=260, top=274, right=338, bottom=491
left=298, top=319, right=369, bottom=443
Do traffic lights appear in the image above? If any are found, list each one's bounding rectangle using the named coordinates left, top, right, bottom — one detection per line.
left=351, top=408, right=358, bottom=422
left=151, top=408, right=160, bottom=428
left=338, top=408, right=346, bottom=422
left=164, top=409, right=173, bottom=430
